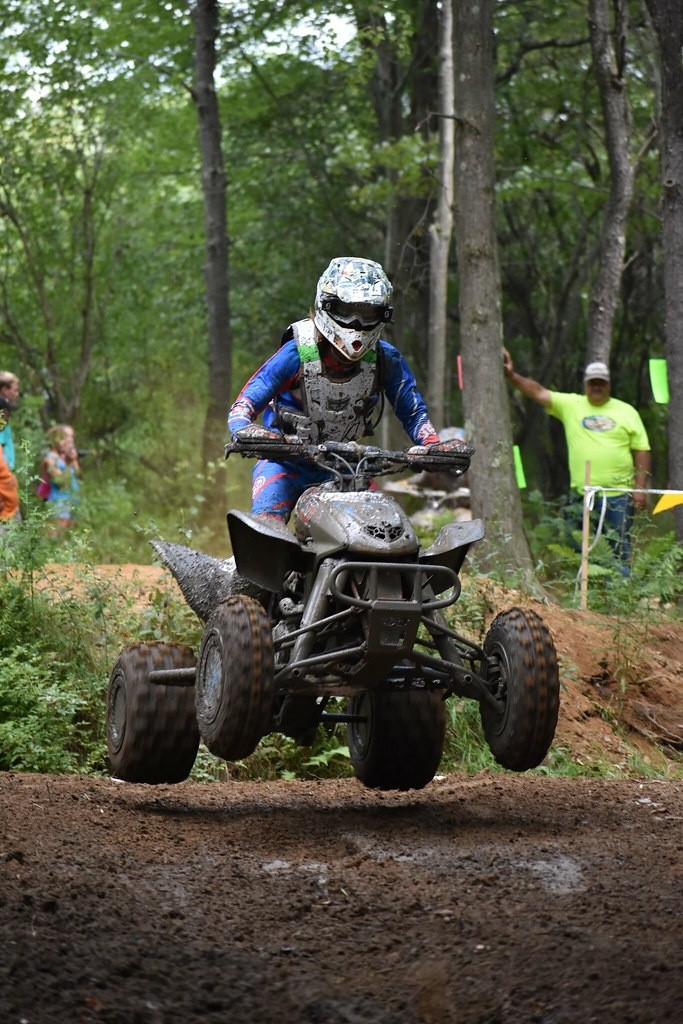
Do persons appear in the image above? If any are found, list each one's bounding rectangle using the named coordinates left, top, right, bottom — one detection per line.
left=0, top=369, right=86, bottom=548
left=501, top=346, right=651, bottom=582
left=227, top=258, right=475, bottom=526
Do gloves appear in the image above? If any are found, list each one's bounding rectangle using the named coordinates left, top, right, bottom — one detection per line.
left=231, top=425, right=304, bottom=466
left=424, top=438, right=475, bottom=477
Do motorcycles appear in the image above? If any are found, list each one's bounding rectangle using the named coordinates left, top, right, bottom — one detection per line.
left=105, top=437, right=562, bottom=790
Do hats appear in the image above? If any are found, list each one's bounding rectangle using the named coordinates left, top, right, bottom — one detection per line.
left=583, top=363, right=612, bottom=383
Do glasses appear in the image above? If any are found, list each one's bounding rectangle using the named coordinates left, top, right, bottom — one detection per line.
left=587, top=379, right=607, bottom=388
left=320, top=299, right=391, bottom=332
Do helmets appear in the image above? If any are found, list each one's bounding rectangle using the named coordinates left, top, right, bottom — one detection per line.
left=312, top=252, right=393, bottom=358
